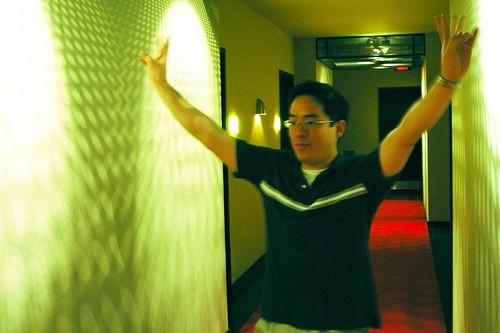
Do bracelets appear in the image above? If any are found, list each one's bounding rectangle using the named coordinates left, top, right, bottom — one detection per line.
left=437, top=75, right=463, bottom=89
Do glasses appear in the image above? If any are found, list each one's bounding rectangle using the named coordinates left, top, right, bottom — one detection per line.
left=283, top=120, right=342, bottom=136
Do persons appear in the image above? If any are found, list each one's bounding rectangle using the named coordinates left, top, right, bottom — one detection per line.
left=137, top=13, right=479, bottom=333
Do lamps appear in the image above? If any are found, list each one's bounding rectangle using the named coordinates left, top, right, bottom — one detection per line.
left=256, top=98, right=266, bottom=113
